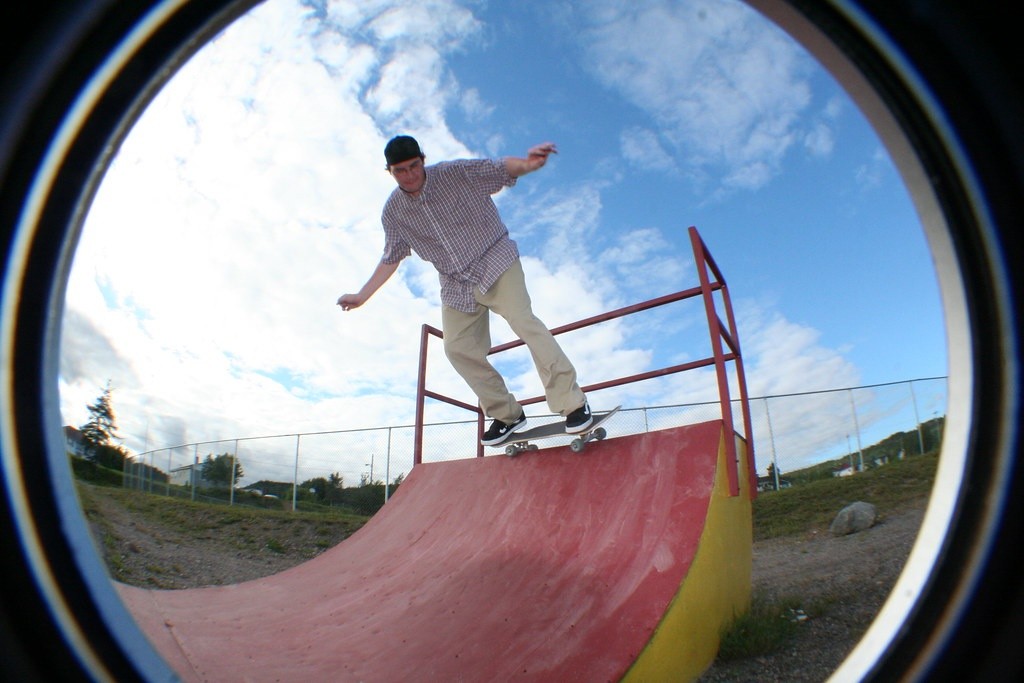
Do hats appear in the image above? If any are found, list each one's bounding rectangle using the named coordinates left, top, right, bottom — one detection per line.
left=384, top=136, right=421, bottom=166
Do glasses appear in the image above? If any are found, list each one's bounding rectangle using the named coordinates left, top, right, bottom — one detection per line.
left=389, top=158, right=421, bottom=176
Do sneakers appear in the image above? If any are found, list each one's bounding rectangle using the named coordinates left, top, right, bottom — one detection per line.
left=564, top=400, right=594, bottom=434
left=481, top=409, right=527, bottom=446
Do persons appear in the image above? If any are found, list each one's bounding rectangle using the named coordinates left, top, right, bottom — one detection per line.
left=335, top=135, right=593, bottom=446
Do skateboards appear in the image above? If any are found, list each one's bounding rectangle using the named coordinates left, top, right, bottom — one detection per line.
left=490, top=404, right=624, bottom=456
